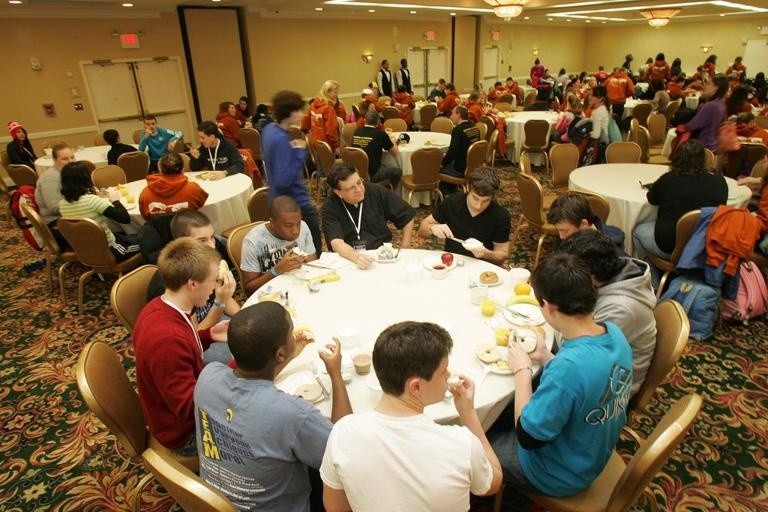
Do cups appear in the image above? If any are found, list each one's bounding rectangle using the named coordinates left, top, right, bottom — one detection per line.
left=506, top=266, right=532, bottom=289
left=259, top=292, right=281, bottom=304
left=42, top=148, right=54, bottom=156
left=116, top=185, right=135, bottom=204
left=431, top=263, right=447, bottom=280
left=350, top=351, right=374, bottom=377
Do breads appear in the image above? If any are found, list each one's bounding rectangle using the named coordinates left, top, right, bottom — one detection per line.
left=216, top=260, right=230, bottom=280
left=292, top=246, right=308, bottom=257
left=462, top=237, right=484, bottom=251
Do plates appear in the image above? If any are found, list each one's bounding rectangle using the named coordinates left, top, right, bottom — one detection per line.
left=476, top=272, right=503, bottom=286
left=422, top=255, right=458, bottom=271
left=293, top=382, right=323, bottom=402
left=504, top=303, right=548, bottom=327
left=123, top=202, right=138, bottom=210
left=477, top=345, right=524, bottom=376
left=294, top=264, right=334, bottom=281
left=370, top=250, right=400, bottom=262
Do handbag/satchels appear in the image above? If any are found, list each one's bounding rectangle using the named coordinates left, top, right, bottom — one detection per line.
left=719, top=120, right=739, bottom=154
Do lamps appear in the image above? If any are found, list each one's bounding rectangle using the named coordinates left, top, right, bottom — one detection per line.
left=112, top=30, right=144, bottom=49
left=490, top=29, right=504, bottom=40
left=639, top=8, right=682, bottom=29
left=756, top=25, right=768, bottom=35
left=532, top=49, right=539, bottom=56
left=362, top=54, right=373, bottom=63
left=701, top=46, right=713, bottom=53
left=424, top=31, right=435, bottom=41
left=482, top=0, right=531, bottom=21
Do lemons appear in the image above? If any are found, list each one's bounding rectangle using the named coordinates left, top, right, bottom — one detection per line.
left=127, top=195, right=135, bottom=203
left=495, top=328, right=511, bottom=346
left=514, top=283, right=531, bottom=295
left=122, top=190, right=128, bottom=196
left=481, top=301, right=496, bottom=317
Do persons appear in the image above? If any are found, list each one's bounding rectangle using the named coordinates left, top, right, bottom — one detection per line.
left=10, top=55, right=768, bottom=512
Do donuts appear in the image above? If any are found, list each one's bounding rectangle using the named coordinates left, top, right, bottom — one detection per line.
left=295, top=384, right=322, bottom=400
left=477, top=345, right=500, bottom=362
left=508, top=328, right=537, bottom=353
left=480, top=271, right=498, bottom=284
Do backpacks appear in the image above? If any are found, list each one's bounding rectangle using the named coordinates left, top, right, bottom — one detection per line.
left=720, top=259, right=768, bottom=321
left=663, top=278, right=719, bottom=341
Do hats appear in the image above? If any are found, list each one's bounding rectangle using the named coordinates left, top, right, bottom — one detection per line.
left=8, top=121, right=22, bottom=135
left=399, top=133, right=409, bottom=143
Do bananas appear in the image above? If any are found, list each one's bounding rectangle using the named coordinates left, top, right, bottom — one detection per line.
left=308, top=273, right=341, bottom=291
left=505, top=296, right=540, bottom=307
left=114, top=185, right=125, bottom=191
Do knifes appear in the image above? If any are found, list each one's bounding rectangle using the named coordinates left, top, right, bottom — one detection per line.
left=304, top=263, right=332, bottom=270
left=448, top=236, right=466, bottom=243
left=395, top=245, right=402, bottom=259
left=500, top=305, right=531, bottom=319
left=312, top=369, right=329, bottom=399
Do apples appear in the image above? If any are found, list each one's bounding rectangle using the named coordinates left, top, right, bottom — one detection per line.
left=441, top=254, right=453, bottom=265
